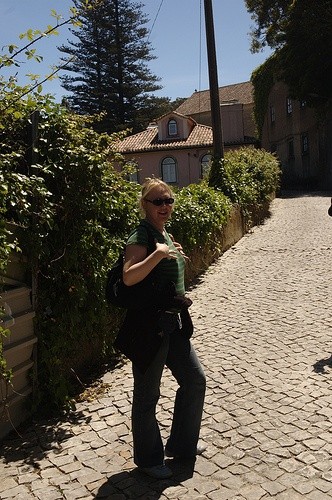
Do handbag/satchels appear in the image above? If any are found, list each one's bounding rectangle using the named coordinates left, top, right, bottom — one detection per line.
left=105, top=225, right=158, bottom=306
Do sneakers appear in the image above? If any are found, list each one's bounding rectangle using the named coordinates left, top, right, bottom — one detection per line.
left=139, top=464, right=172, bottom=479
left=165, top=446, right=205, bottom=456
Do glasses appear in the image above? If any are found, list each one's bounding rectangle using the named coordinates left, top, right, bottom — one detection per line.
left=143, top=197, right=174, bottom=205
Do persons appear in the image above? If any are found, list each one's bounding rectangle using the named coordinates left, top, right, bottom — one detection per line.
left=118, top=176, right=210, bottom=479
